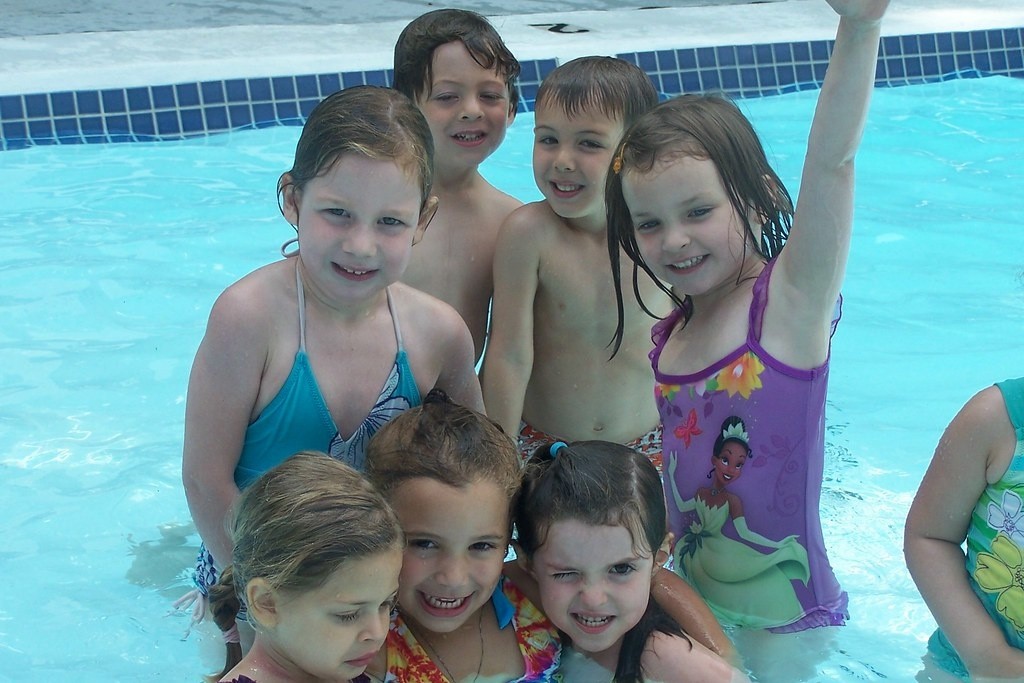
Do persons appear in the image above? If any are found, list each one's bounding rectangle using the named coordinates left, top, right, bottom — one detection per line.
left=508, top=437, right=755, bottom=683
left=904, top=376, right=1024, bottom=683
left=364, top=387, right=736, bottom=683
left=480, top=54, right=685, bottom=477
left=182, top=84, right=487, bottom=627
left=391, top=7, right=527, bottom=368
left=603, top=0, right=891, bottom=683
left=203, top=449, right=409, bottom=683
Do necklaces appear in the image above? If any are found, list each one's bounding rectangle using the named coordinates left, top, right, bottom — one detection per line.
left=401, top=604, right=486, bottom=683
